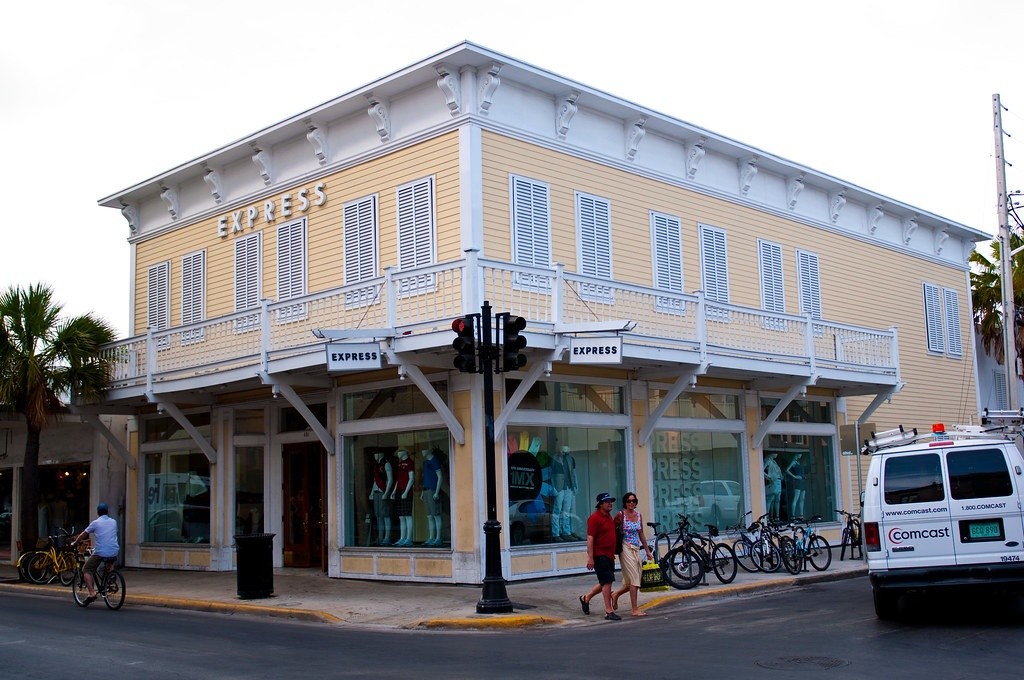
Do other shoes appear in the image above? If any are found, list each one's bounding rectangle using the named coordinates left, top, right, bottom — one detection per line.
left=430, top=537, right=442, bottom=546
left=404, top=538, right=413, bottom=546
left=561, top=534, right=578, bottom=542
left=551, top=536, right=564, bottom=543
left=422, top=537, right=434, bottom=546
left=379, top=537, right=390, bottom=547
left=393, top=538, right=405, bottom=546
left=372, top=538, right=383, bottom=546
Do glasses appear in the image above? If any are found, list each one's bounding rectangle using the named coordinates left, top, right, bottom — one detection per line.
left=625, top=499, right=638, bottom=504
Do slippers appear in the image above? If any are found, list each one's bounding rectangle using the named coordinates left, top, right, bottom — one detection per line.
left=82, top=594, right=97, bottom=603
left=611, top=591, right=618, bottom=610
left=633, top=611, right=647, bottom=616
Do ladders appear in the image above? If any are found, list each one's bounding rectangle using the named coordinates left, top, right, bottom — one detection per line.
left=864, top=425, right=917, bottom=448
left=981, top=407, right=1024, bottom=428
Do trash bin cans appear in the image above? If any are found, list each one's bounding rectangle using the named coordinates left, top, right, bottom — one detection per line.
left=233, top=533, right=277, bottom=599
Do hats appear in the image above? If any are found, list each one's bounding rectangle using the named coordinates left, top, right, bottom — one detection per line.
left=394, top=447, right=411, bottom=456
left=594, top=493, right=615, bottom=508
left=371, top=448, right=384, bottom=457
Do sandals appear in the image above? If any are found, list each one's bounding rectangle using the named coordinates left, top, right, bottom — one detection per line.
left=580, top=595, right=590, bottom=615
left=605, top=611, right=621, bottom=620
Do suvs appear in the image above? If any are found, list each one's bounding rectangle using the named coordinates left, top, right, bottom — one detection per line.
left=860, top=424, right=1024, bottom=620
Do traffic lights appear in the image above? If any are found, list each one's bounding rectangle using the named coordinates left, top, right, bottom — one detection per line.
left=503, top=312, right=527, bottom=369
left=451, top=318, right=476, bottom=372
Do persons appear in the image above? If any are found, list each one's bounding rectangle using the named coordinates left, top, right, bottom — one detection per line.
left=551, top=445, right=578, bottom=541
left=369, top=452, right=393, bottom=543
left=611, top=492, right=653, bottom=616
left=786, top=452, right=806, bottom=522
left=579, top=492, right=622, bottom=620
left=763, top=453, right=784, bottom=524
left=70, top=502, right=120, bottom=603
left=420, top=449, right=443, bottom=545
left=390, top=450, right=414, bottom=546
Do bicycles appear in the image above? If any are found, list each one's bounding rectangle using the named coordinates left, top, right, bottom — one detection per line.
left=72, top=552, right=126, bottom=611
left=725, top=510, right=832, bottom=575
left=834, top=509, right=862, bottom=561
left=16, top=524, right=77, bottom=587
left=640, top=513, right=738, bottom=590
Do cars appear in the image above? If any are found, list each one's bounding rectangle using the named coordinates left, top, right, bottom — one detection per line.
left=697, top=480, right=740, bottom=522
left=148, top=505, right=210, bottom=542
left=509, top=499, right=582, bottom=545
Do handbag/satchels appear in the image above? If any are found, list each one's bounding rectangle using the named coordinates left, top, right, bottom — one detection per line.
left=641, top=555, right=669, bottom=592
left=615, top=510, right=626, bottom=554
